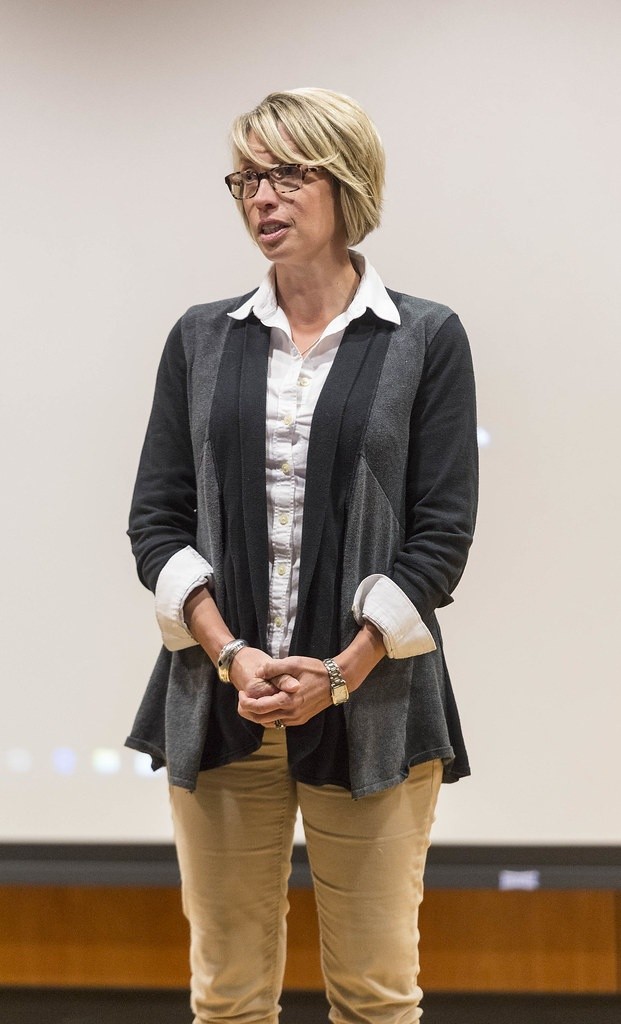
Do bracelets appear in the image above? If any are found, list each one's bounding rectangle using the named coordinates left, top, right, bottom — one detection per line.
left=217, top=637, right=248, bottom=684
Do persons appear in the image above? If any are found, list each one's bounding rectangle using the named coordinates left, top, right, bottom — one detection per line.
left=124, top=85, right=480, bottom=1023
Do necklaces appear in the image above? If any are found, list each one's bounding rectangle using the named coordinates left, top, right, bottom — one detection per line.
left=298, top=336, right=319, bottom=355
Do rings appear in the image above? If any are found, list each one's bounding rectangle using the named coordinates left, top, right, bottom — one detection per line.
left=275, top=719, right=286, bottom=730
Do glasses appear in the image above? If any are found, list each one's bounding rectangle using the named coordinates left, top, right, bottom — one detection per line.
left=225, top=164, right=325, bottom=199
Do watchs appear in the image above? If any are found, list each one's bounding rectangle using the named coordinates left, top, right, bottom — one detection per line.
left=322, top=658, right=350, bottom=706
